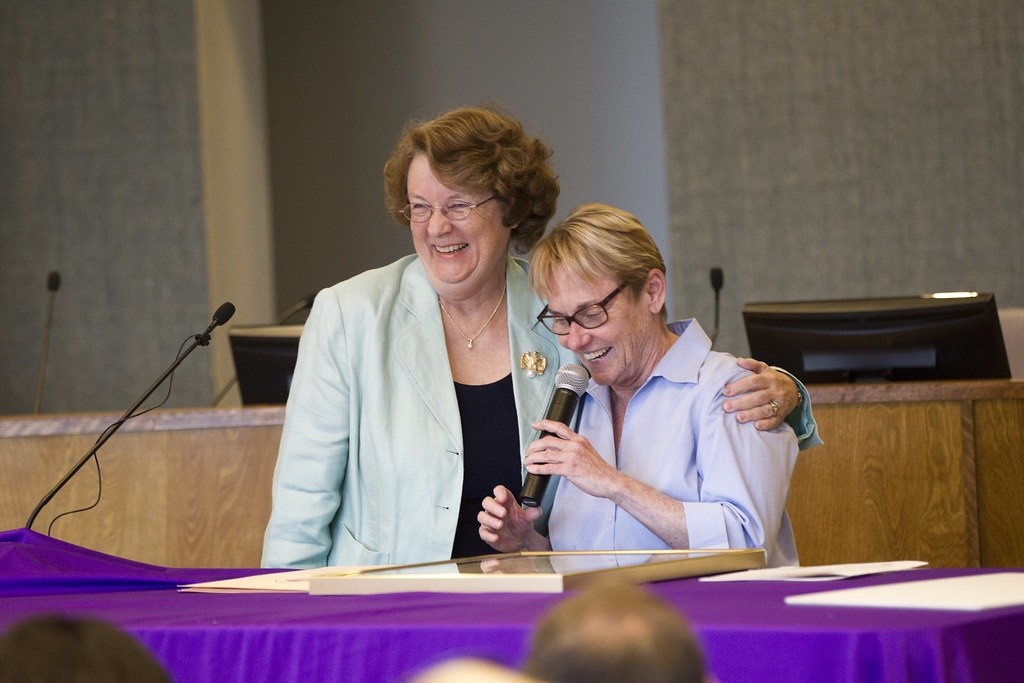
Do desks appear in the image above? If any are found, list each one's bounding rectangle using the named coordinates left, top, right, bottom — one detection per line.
left=0, top=523, right=1024, bottom=683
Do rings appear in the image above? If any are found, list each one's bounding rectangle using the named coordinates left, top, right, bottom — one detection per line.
left=770, top=401, right=779, bottom=416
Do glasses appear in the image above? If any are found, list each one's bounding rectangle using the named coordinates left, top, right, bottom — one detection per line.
left=536, top=284, right=626, bottom=336
left=399, top=195, right=496, bottom=223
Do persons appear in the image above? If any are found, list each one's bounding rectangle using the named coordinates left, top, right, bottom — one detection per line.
left=260, top=107, right=825, bottom=569
left=527, top=584, right=706, bottom=683
left=476, top=203, right=800, bottom=568
left=0, top=611, right=170, bottom=683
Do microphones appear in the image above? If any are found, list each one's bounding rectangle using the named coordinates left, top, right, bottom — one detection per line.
left=32, top=270, right=61, bottom=413
left=28, top=301, right=238, bottom=532
left=709, top=266, right=723, bottom=348
left=212, top=293, right=318, bottom=406
left=519, top=363, right=590, bottom=509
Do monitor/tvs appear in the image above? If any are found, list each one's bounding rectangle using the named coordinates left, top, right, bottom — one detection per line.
left=742, top=293, right=1013, bottom=386
left=227, top=321, right=304, bottom=406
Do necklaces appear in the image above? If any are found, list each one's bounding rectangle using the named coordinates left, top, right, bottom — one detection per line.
left=439, top=282, right=506, bottom=349
